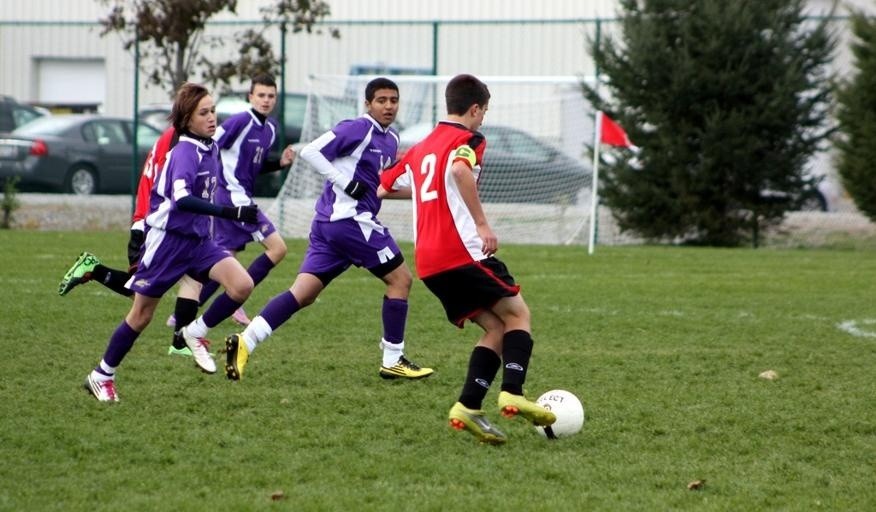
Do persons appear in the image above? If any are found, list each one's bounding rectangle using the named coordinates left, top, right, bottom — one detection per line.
left=167, top=76, right=295, bottom=327
left=58, top=125, right=216, bottom=358
left=84, top=85, right=255, bottom=403
left=375, top=75, right=556, bottom=443
left=226, top=76, right=435, bottom=382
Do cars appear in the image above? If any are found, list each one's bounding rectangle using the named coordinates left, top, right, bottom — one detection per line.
left=763, top=153, right=855, bottom=217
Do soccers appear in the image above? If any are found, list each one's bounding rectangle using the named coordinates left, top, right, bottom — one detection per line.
left=534, top=389, right=584, bottom=438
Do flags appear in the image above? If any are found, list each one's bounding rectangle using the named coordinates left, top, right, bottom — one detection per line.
left=599, top=112, right=633, bottom=148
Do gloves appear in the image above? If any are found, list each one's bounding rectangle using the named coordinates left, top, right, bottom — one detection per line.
left=221, top=205, right=258, bottom=224
left=345, top=180, right=368, bottom=201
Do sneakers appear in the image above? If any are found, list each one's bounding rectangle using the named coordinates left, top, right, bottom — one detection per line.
left=379, top=355, right=434, bottom=380
left=447, top=401, right=507, bottom=446
left=225, top=332, right=250, bottom=380
left=82, top=370, right=120, bottom=402
left=175, top=323, right=217, bottom=373
left=497, top=390, right=556, bottom=426
left=168, top=346, right=216, bottom=360
left=58, top=251, right=99, bottom=296
left=233, top=308, right=250, bottom=325
